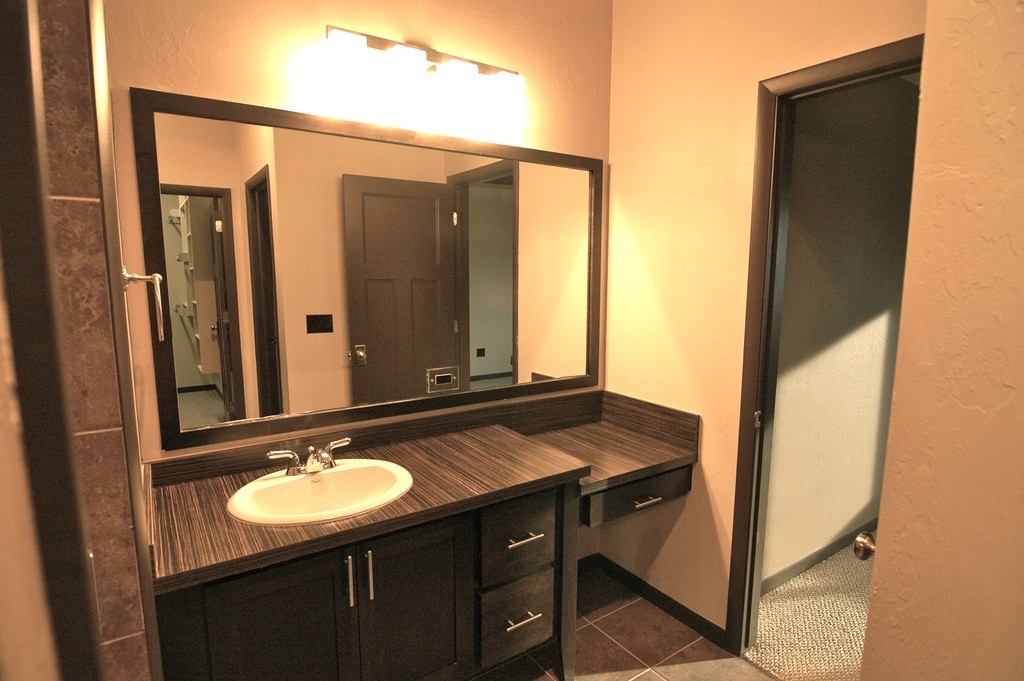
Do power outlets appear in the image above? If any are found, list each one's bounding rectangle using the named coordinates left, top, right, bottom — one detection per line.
left=476, top=347, right=485, bottom=357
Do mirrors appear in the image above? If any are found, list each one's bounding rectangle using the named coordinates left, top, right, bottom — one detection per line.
left=130, top=85, right=603, bottom=451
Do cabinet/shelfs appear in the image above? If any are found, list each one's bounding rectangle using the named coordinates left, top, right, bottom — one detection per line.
left=176, top=193, right=221, bottom=375
left=473, top=482, right=561, bottom=681
left=203, top=512, right=477, bottom=680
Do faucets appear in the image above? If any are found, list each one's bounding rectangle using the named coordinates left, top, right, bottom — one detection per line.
left=304, top=448, right=330, bottom=474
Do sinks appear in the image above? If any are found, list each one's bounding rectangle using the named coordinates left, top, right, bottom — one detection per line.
left=226, top=458, right=414, bottom=527
